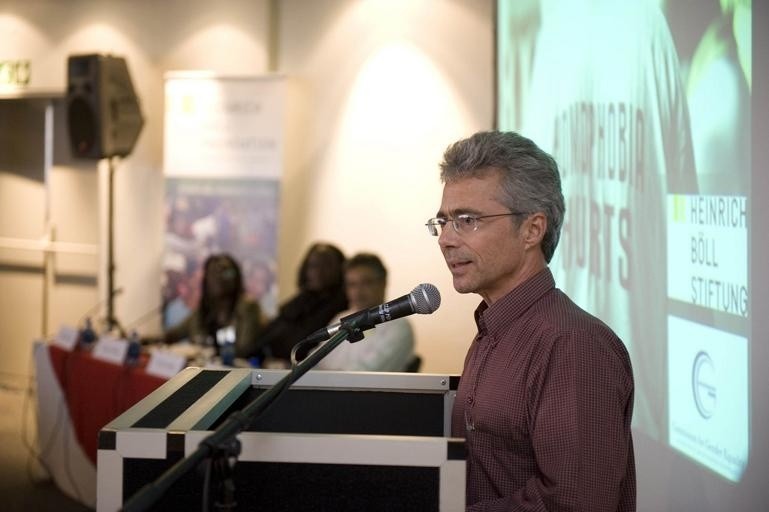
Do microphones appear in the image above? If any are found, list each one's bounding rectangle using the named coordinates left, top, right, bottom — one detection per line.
left=307, top=283, right=441, bottom=343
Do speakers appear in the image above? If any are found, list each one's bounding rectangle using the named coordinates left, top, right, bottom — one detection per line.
left=66, top=54, right=144, bottom=161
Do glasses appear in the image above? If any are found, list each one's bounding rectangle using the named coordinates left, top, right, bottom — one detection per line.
left=424, top=212, right=523, bottom=236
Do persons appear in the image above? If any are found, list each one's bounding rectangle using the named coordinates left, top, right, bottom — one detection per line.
left=164, top=178, right=277, bottom=328
left=260, top=243, right=346, bottom=370
left=316, top=251, right=415, bottom=371
left=437, top=130, right=637, bottom=512
left=138, top=251, right=264, bottom=362
